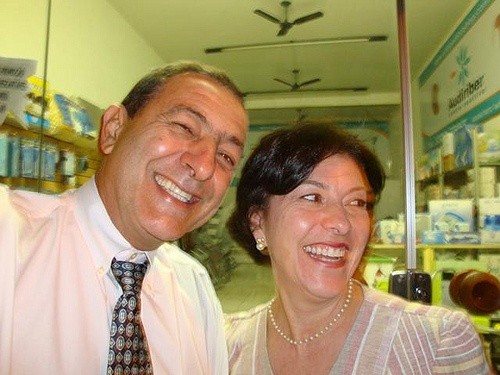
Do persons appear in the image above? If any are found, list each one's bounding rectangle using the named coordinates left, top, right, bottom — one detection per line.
left=0, top=59, right=250, bottom=375
left=223, top=121, right=492, bottom=375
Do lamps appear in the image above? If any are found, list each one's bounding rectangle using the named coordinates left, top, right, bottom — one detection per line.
left=205, top=36, right=387, bottom=54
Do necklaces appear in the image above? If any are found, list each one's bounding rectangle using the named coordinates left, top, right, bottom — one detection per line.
left=268, top=278, right=354, bottom=346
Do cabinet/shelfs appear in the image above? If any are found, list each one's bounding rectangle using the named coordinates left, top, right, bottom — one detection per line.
left=353, top=125, right=500, bottom=375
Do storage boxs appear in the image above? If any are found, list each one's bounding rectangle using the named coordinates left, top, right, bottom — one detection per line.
left=428, top=199, right=474, bottom=234
left=477, top=197, right=500, bottom=245
left=360, top=256, right=394, bottom=294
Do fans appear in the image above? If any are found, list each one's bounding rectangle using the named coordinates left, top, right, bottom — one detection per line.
left=254, top=1, right=323, bottom=37
left=274, top=69, right=320, bottom=92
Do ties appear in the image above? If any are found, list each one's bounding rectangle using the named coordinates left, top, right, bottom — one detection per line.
left=106, top=257, right=154, bottom=375
